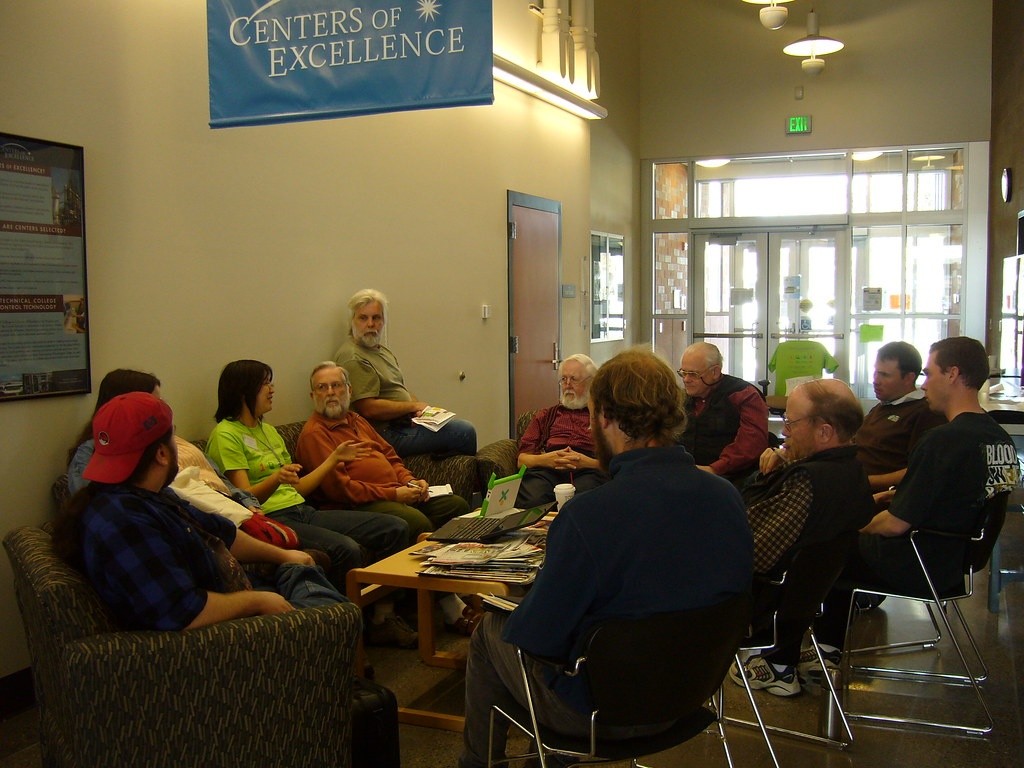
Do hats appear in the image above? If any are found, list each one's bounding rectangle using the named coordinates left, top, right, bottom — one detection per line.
left=81, top=391, right=173, bottom=484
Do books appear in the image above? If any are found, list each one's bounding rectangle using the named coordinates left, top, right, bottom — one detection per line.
left=408, top=542, right=545, bottom=585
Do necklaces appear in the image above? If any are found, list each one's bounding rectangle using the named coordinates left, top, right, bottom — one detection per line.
left=238, top=416, right=283, bottom=469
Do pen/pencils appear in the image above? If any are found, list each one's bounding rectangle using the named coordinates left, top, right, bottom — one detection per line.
left=407, top=482, right=434, bottom=494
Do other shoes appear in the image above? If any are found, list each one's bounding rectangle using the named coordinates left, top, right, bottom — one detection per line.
left=369, top=615, right=418, bottom=646
left=445, top=606, right=480, bottom=635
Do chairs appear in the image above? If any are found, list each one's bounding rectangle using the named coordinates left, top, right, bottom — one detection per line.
left=841, top=486, right=1013, bottom=735
left=478, top=410, right=547, bottom=506
left=488, top=610, right=747, bottom=768
left=717, top=524, right=855, bottom=754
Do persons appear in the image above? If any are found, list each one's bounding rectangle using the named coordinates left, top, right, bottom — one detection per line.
left=50, top=289, right=477, bottom=648
left=466, top=320, right=1024, bottom=768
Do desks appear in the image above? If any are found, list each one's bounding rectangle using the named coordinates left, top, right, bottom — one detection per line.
left=976, top=381, right=1024, bottom=612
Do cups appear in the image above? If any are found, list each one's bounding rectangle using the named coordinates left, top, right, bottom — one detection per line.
left=554, top=484, right=576, bottom=512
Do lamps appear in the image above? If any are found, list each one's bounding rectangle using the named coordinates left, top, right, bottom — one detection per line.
left=783, top=9, right=844, bottom=76
left=742, top=0, right=794, bottom=30
left=494, top=53, right=609, bottom=122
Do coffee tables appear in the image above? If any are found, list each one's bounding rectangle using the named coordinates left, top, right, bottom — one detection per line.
left=346, top=507, right=559, bottom=733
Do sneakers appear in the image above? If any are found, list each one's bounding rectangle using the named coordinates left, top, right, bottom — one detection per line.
left=729, top=652, right=803, bottom=696
left=801, top=644, right=843, bottom=677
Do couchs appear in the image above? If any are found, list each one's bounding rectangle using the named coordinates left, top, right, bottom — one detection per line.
left=0, top=527, right=401, bottom=768
left=48, top=418, right=477, bottom=529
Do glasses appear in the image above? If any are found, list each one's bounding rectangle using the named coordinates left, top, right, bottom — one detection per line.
left=557, top=375, right=591, bottom=388
left=781, top=413, right=825, bottom=432
left=676, top=364, right=718, bottom=378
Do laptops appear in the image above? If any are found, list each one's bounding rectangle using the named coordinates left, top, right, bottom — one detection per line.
left=460, top=464, right=528, bottom=518
left=426, top=501, right=560, bottom=543
